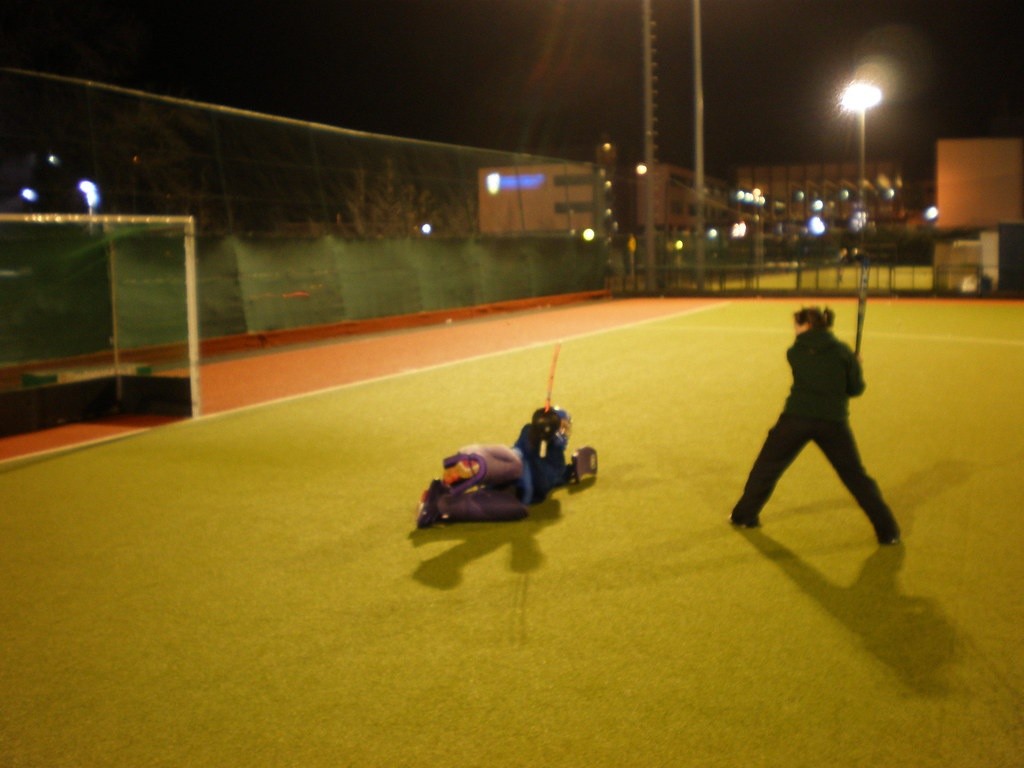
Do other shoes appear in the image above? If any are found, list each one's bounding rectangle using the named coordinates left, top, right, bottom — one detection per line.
left=876, top=525, right=899, bottom=543
left=728, top=511, right=761, bottom=527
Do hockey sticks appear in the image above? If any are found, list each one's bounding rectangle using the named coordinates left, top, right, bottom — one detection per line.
left=854, top=253, right=869, bottom=354
left=539, top=343, right=562, bottom=459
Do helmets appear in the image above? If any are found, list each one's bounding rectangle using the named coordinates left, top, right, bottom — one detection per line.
left=552, top=407, right=572, bottom=449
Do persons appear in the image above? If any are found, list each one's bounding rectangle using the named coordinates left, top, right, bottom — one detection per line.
left=728, top=307, right=900, bottom=544
left=415, top=406, right=598, bottom=530
